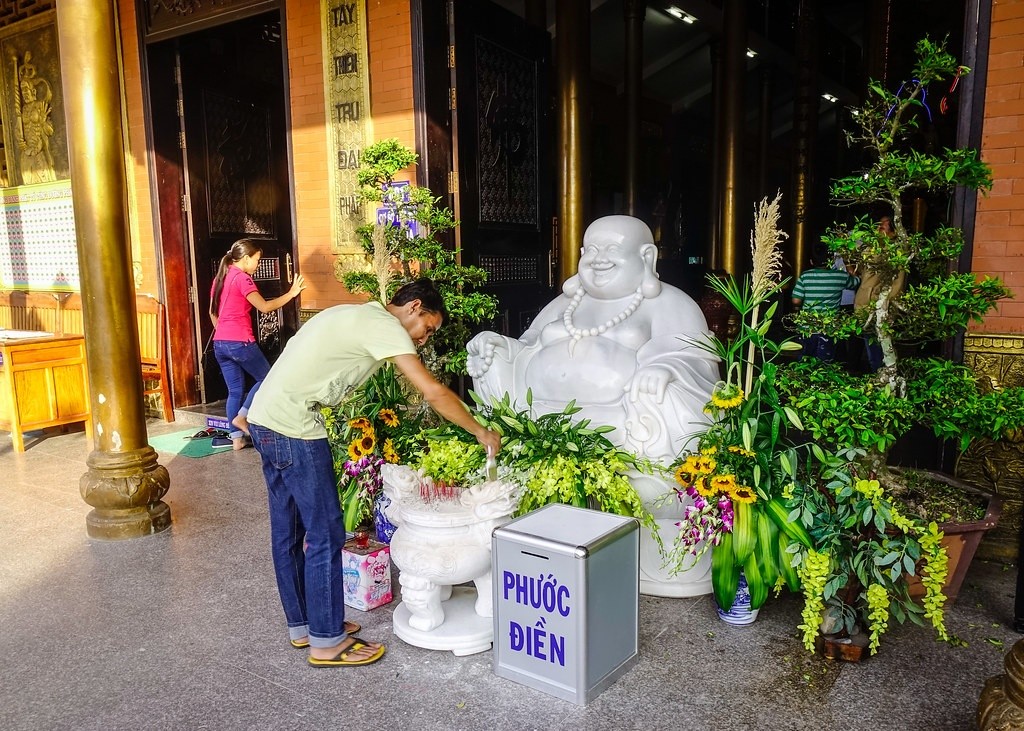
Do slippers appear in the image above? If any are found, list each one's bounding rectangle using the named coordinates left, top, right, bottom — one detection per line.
left=307, top=636, right=385, bottom=669
left=290, top=620, right=361, bottom=650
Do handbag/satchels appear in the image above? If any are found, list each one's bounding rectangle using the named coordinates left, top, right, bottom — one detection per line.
left=201, top=348, right=220, bottom=371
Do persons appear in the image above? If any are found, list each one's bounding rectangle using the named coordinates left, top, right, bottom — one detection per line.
left=465, top=215, right=723, bottom=519
left=209, top=238, right=307, bottom=451
left=247, top=277, right=501, bottom=668
left=792, top=216, right=896, bottom=372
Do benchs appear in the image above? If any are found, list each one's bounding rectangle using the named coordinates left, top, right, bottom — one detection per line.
left=0, top=291, right=175, bottom=423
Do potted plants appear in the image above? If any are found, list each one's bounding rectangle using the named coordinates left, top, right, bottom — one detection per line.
left=324, top=38, right=1024, bottom=662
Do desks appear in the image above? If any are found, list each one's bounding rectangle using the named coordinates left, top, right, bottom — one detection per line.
left=1, top=329, right=93, bottom=451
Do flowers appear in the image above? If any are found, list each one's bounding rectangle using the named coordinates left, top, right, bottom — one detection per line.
left=659, top=362, right=758, bottom=579
left=404, top=387, right=664, bottom=554
left=321, top=398, right=400, bottom=529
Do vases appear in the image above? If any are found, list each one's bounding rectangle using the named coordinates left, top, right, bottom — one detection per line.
left=374, top=484, right=397, bottom=542
left=712, top=566, right=760, bottom=625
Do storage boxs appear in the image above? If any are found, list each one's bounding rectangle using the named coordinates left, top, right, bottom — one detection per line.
left=491, top=501, right=640, bottom=703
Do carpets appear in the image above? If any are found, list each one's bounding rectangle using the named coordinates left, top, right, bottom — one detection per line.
left=147, top=426, right=235, bottom=458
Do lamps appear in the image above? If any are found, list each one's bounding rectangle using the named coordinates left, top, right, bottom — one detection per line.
left=664, top=4, right=699, bottom=24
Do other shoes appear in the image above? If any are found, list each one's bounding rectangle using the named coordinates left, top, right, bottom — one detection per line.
left=211, top=437, right=233, bottom=449
left=192, top=427, right=215, bottom=440
left=210, top=430, right=231, bottom=439
